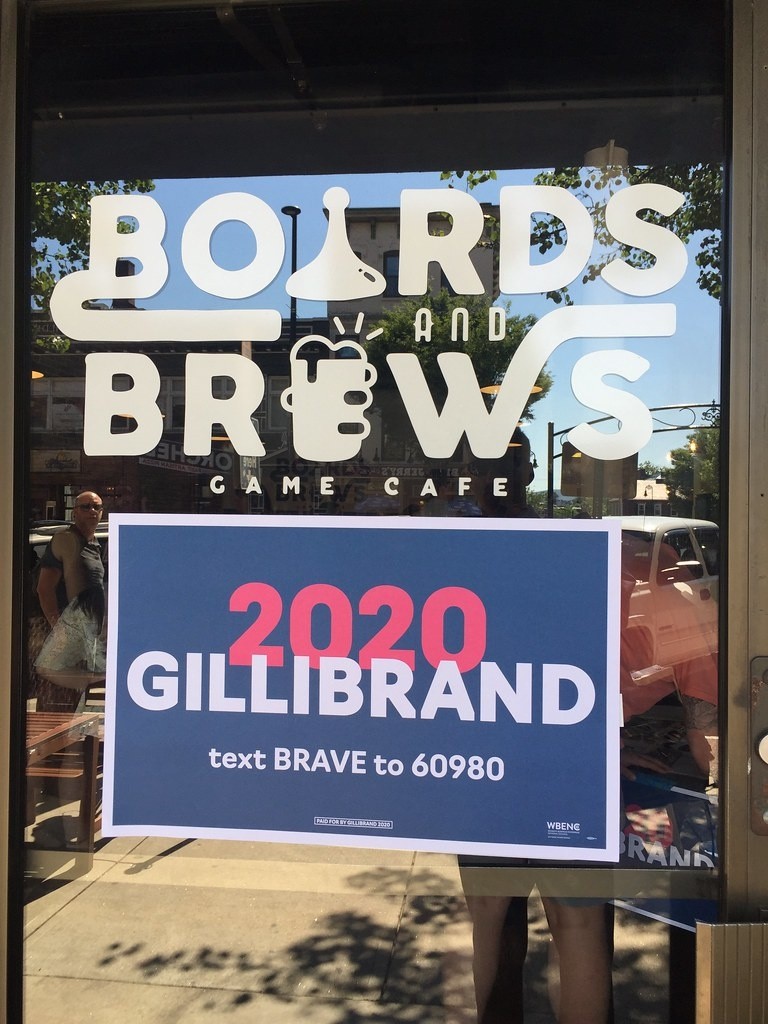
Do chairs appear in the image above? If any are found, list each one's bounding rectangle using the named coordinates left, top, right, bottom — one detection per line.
left=27, top=681, right=106, bottom=822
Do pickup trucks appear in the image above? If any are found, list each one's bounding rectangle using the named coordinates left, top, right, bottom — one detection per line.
left=606, top=516, right=721, bottom=685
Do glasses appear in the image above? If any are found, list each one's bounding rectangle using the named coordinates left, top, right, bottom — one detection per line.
left=74, top=502, right=104, bottom=512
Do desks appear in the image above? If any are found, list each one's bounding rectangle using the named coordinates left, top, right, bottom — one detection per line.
left=26, top=712, right=100, bottom=853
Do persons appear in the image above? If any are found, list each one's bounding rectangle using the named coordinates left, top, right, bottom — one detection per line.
left=34, top=492, right=105, bottom=704
left=620, top=529, right=719, bottom=836
left=33, top=569, right=108, bottom=850
left=456, top=426, right=614, bottom=1024
